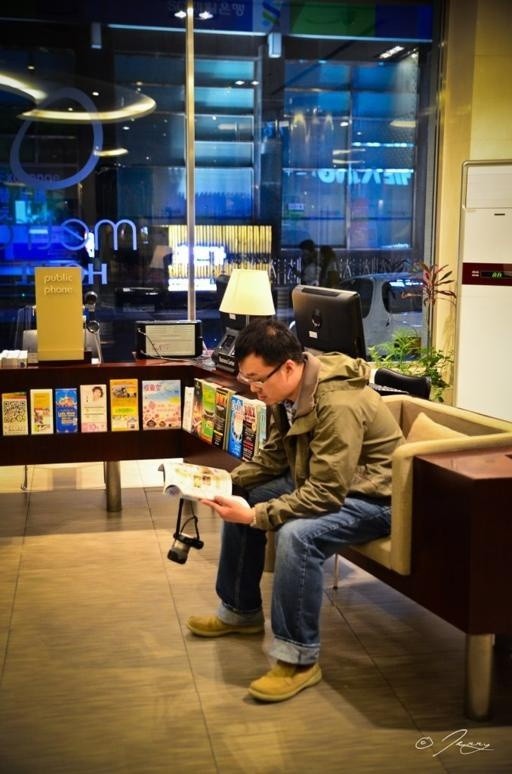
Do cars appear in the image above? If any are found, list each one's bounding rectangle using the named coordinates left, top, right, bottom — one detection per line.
left=288, top=271, right=424, bottom=361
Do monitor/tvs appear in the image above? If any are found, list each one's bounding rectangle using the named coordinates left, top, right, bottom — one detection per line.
left=291, top=284, right=366, bottom=359
left=211, top=274, right=278, bottom=374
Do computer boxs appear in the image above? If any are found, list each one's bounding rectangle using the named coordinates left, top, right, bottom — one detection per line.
left=135, top=319, right=202, bottom=358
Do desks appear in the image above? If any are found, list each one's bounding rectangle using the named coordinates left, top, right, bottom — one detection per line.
left=0, top=350, right=256, bottom=513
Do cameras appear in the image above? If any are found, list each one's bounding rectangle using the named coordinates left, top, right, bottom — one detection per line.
left=167, top=530, right=204, bottom=565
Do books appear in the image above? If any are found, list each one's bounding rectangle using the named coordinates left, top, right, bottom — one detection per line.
left=0, top=377, right=268, bottom=464
left=157, top=461, right=251, bottom=512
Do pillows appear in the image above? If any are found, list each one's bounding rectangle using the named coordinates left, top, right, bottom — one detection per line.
left=408, top=411, right=468, bottom=441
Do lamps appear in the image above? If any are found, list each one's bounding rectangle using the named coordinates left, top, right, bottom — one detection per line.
left=219, top=267, right=279, bottom=330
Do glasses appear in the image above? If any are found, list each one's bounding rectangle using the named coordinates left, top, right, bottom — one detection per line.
left=236, top=362, right=282, bottom=390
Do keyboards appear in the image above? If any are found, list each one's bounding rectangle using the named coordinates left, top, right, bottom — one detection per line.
left=368, top=381, right=409, bottom=397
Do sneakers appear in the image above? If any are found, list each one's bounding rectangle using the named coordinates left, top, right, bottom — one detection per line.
left=186, top=615, right=264, bottom=637
left=249, top=659, right=323, bottom=703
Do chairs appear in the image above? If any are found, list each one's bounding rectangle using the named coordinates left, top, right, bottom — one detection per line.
left=15, top=305, right=107, bottom=492
left=262, top=394, right=511, bottom=720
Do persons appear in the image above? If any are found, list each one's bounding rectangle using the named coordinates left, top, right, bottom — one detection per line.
left=184, top=317, right=407, bottom=705
